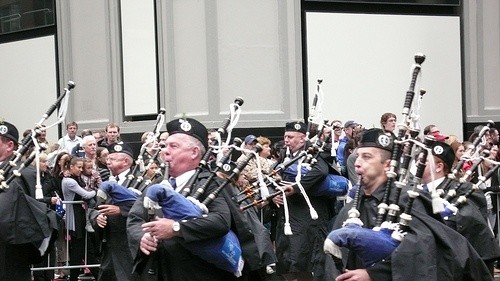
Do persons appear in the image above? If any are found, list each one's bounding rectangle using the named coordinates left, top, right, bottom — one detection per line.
left=127, top=118, right=231, bottom=281
left=0, top=121, right=32, bottom=281
left=23, top=112, right=500, bottom=281
left=325, top=130, right=438, bottom=281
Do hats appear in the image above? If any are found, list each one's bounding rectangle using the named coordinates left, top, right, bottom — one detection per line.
left=285, top=121, right=307, bottom=135
left=357, top=128, right=397, bottom=153
left=0, top=121, right=19, bottom=151
left=107, top=141, right=135, bottom=158
left=430, top=141, right=455, bottom=168
left=343, top=120, right=356, bottom=131
left=245, top=135, right=259, bottom=145
left=79, top=135, right=95, bottom=147
left=166, top=118, right=208, bottom=152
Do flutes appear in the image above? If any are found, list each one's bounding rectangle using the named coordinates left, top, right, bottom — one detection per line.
left=92, top=106, right=168, bottom=245
left=142, top=52, right=500, bottom=281
left=0, top=79, right=77, bottom=195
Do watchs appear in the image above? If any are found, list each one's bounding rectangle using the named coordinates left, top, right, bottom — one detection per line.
left=172, top=220, right=181, bottom=233
